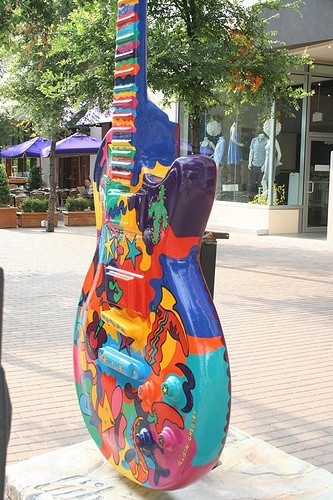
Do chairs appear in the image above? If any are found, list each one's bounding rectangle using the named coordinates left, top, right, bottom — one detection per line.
left=9, top=189, right=93, bottom=207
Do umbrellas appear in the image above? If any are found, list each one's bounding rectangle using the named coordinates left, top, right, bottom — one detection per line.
left=40, top=128, right=102, bottom=185
left=0, top=137, right=52, bottom=190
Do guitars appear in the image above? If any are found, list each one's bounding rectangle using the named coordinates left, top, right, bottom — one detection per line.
left=73, top=0, right=232, bottom=491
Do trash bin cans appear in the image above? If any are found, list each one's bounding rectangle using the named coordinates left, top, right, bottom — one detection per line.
left=200, top=231, right=229, bottom=301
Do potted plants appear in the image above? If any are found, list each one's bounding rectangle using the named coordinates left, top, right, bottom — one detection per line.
left=0, top=162, right=17, bottom=228
left=62, top=194, right=97, bottom=226
left=16, top=198, right=58, bottom=227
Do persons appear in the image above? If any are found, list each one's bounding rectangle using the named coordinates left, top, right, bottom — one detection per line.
left=198, top=121, right=283, bottom=205
left=81, top=177, right=94, bottom=195
